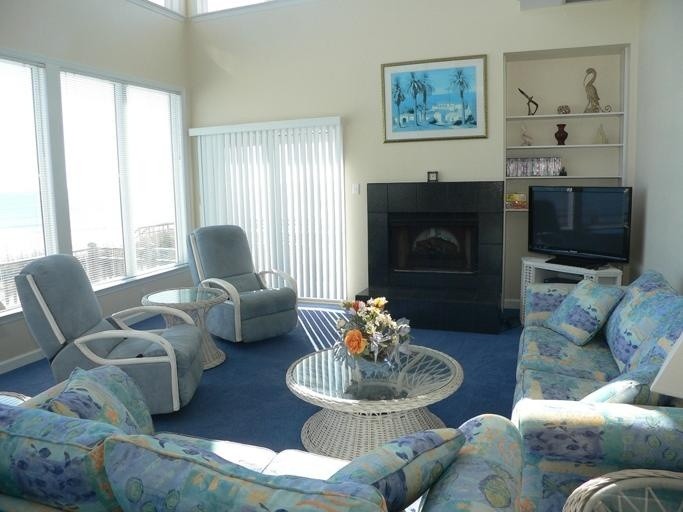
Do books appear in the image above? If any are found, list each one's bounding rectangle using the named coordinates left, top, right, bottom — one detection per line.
left=506, top=156, right=561, bottom=177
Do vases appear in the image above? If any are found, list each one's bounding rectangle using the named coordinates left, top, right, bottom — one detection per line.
left=552, top=123, right=568, bottom=144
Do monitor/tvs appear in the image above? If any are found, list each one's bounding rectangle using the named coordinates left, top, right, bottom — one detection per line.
left=528, top=186, right=632, bottom=271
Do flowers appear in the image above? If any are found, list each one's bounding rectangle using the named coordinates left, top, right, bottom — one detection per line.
left=331, top=295, right=412, bottom=364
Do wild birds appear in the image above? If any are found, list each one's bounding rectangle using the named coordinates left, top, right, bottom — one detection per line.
left=583, top=68, right=600, bottom=112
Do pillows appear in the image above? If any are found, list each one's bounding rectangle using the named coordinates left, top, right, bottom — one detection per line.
left=33, top=365, right=145, bottom=437
left=540, top=271, right=624, bottom=348
left=325, top=427, right=466, bottom=512
left=577, top=363, right=672, bottom=406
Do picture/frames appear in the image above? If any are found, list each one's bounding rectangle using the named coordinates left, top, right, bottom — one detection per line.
left=379, top=53, right=489, bottom=143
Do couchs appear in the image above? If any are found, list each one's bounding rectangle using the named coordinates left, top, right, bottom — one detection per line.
left=508, top=281, right=682, bottom=512
left=1, top=361, right=524, bottom=512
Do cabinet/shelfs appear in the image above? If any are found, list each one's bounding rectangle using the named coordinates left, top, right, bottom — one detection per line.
left=502, top=47, right=628, bottom=314
left=519, top=255, right=623, bottom=331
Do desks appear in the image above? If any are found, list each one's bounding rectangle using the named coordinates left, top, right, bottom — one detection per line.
left=285, top=343, right=464, bottom=462
left=559, top=468, right=682, bottom=512
left=0, top=390, right=32, bottom=407
left=140, top=286, right=228, bottom=371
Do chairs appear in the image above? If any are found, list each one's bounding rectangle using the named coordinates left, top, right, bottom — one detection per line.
left=14, top=252, right=205, bottom=415
left=185, top=224, right=298, bottom=344
